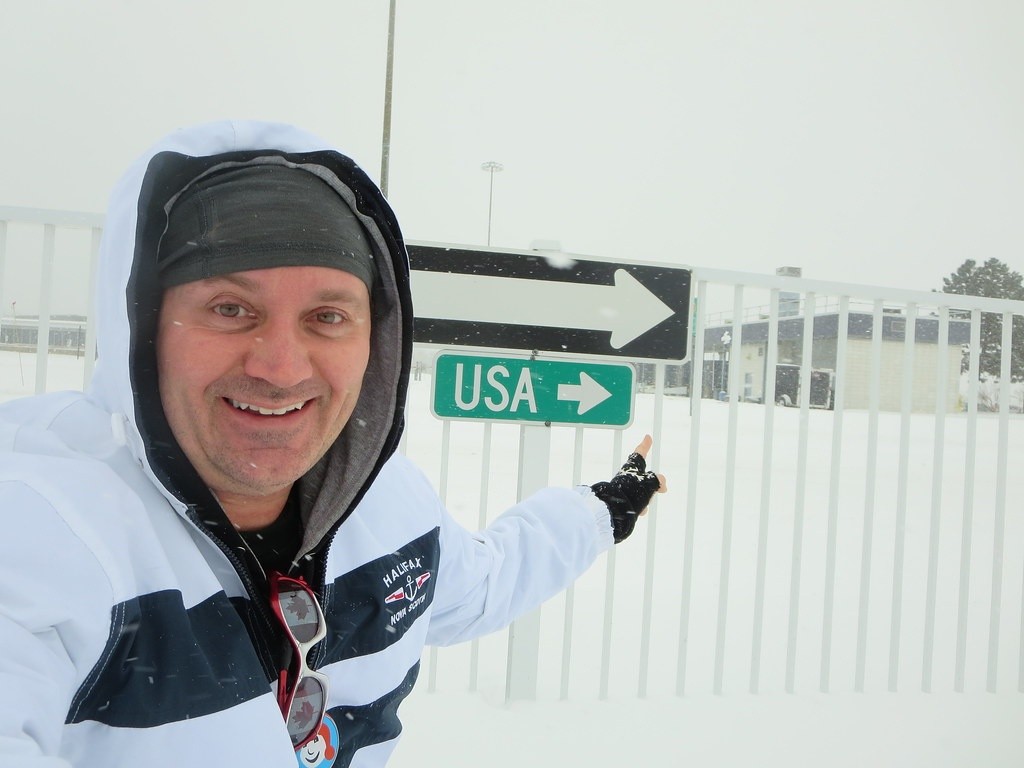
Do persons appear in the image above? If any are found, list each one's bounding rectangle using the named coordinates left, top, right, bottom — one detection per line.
left=1, top=118, right=669, bottom=768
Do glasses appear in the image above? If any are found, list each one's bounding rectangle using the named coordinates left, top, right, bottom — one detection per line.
left=267, top=569, right=331, bottom=752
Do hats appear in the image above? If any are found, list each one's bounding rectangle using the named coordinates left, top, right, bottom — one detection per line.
left=157, top=162, right=380, bottom=308
